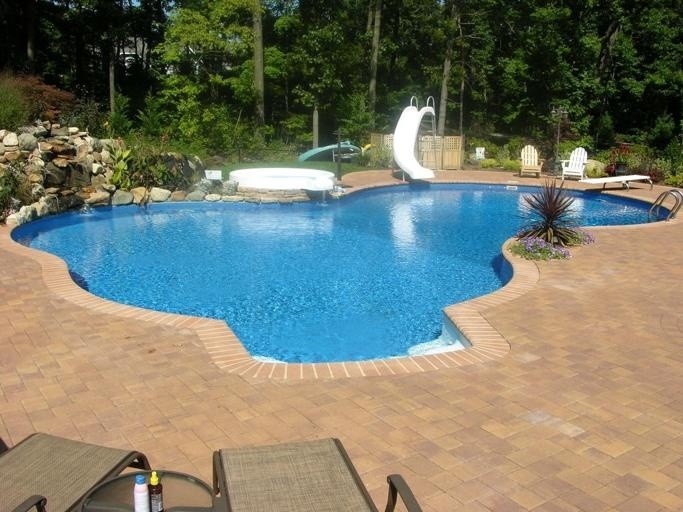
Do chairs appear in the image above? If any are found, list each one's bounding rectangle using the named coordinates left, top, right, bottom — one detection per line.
left=518, top=144, right=544, bottom=177
left=0, top=431, right=152, bottom=512
left=213, top=438, right=423, bottom=512
left=558, top=147, right=589, bottom=181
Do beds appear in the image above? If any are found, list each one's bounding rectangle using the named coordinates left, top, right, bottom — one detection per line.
left=578, top=173, right=654, bottom=191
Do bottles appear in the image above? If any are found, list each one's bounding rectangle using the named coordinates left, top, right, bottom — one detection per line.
left=135, top=472, right=164, bottom=512
